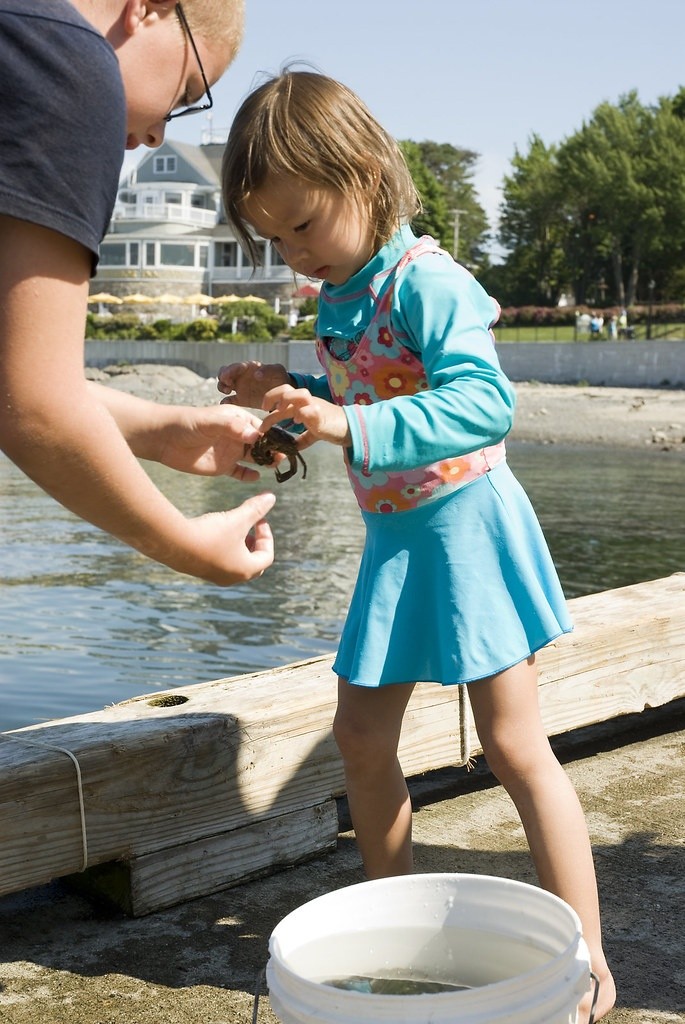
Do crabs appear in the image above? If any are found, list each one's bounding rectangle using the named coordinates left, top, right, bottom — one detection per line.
left=250, top=427, right=308, bottom=483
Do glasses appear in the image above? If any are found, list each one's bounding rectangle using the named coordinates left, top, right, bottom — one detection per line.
left=163, top=1, right=213, bottom=122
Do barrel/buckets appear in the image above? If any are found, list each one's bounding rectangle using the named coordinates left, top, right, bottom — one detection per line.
left=248, top=871, right=602, bottom=1024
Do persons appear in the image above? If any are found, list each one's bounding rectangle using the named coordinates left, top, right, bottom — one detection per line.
left=215, top=62, right=616, bottom=1024
left=0, top=3, right=288, bottom=587
left=583, top=310, right=627, bottom=340
